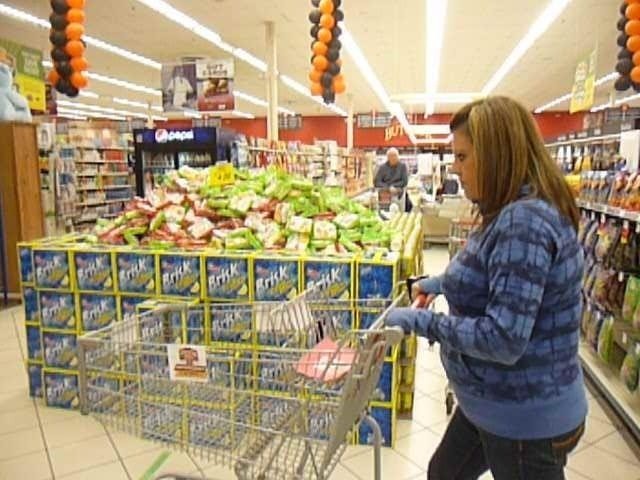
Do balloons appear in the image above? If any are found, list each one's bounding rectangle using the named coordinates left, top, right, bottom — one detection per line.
left=309, top=0, right=346, bottom=105
left=614, top=0, right=640, bottom=93
left=48, top=0, right=89, bottom=97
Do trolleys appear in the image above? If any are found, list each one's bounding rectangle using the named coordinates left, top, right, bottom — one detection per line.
left=447, top=217, right=482, bottom=263
left=75, top=274, right=433, bottom=480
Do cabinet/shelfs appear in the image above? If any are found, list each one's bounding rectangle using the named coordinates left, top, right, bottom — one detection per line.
left=56, top=145, right=134, bottom=234
left=542, top=133, right=640, bottom=440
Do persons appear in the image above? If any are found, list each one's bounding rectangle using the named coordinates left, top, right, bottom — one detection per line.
left=165, top=67, right=194, bottom=106
left=374, top=147, right=408, bottom=189
left=383, top=94, right=588, bottom=480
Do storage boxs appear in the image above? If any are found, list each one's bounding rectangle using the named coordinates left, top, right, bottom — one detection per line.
left=16, top=236, right=415, bottom=448
